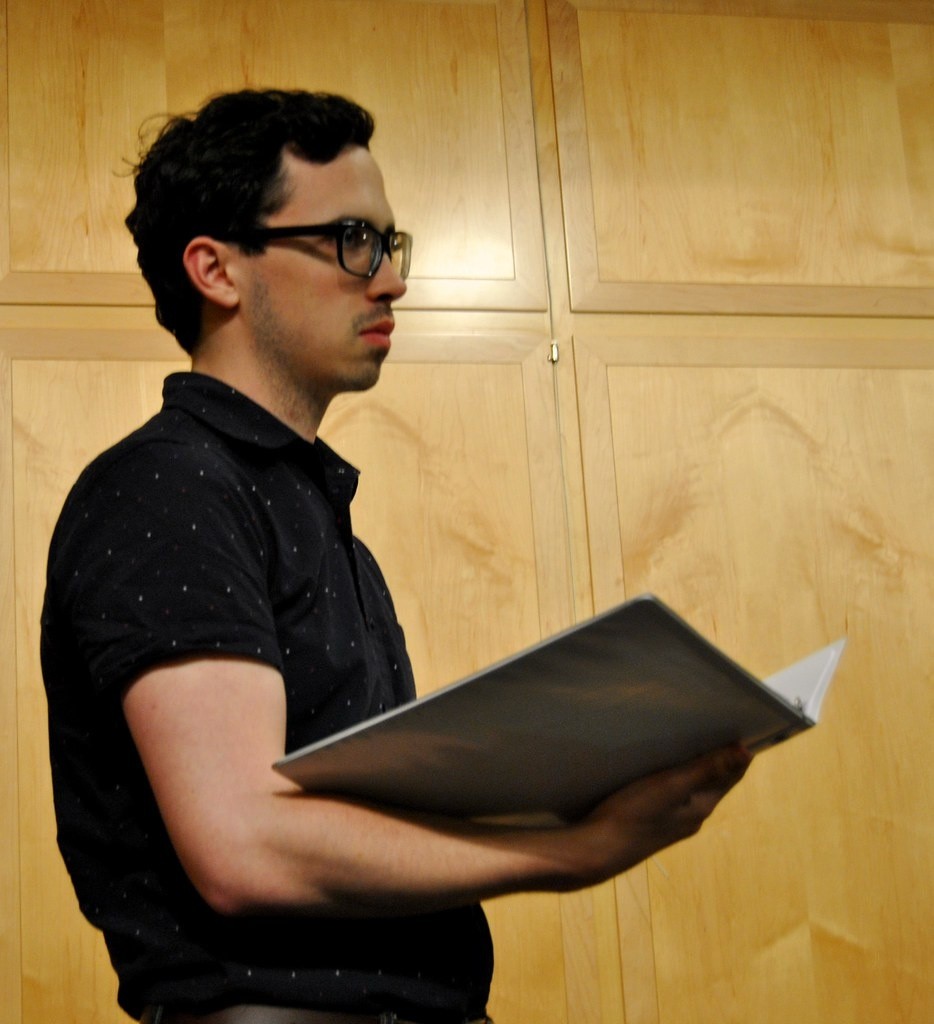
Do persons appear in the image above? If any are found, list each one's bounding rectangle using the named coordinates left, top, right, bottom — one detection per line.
left=40, top=87, right=757, bottom=1024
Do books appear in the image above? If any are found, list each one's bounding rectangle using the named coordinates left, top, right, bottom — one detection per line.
left=273, top=593, right=848, bottom=836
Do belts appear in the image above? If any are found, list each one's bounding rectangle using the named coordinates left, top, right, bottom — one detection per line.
left=140, top=1003, right=496, bottom=1024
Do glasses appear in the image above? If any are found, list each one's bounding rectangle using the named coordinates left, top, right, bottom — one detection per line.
left=217, top=220, right=410, bottom=281
